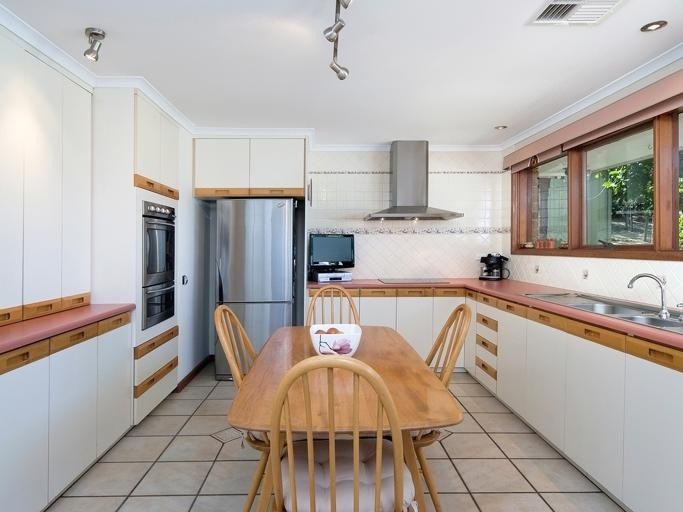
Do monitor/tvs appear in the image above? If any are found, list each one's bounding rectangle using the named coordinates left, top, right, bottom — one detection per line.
left=310, top=234, right=354, bottom=273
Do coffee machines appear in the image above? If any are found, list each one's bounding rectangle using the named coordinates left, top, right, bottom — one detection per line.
left=479, top=253, right=508, bottom=282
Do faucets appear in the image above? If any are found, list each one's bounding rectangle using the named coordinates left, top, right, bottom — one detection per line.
left=628, top=273, right=665, bottom=311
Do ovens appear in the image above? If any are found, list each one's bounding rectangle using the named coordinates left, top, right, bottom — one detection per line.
left=142, top=198, right=176, bottom=331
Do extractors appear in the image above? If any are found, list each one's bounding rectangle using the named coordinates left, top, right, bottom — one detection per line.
left=363, top=141, right=464, bottom=222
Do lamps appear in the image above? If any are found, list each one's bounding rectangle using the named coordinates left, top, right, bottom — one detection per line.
left=324, top=1, right=352, bottom=82
left=84, top=29, right=104, bottom=63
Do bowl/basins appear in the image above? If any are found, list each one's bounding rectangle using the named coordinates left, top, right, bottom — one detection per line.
left=309, top=323, right=362, bottom=357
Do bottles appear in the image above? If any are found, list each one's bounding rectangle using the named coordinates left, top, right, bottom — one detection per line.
left=534, top=239, right=555, bottom=249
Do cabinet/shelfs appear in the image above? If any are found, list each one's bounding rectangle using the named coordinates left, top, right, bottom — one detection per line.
left=477, top=293, right=497, bottom=399
left=464, top=289, right=477, bottom=378
left=133, top=324, right=179, bottom=427
left=0, top=26, right=23, bottom=328
left=0, top=316, right=50, bottom=512
left=566, top=317, right=683, bottom=511
left=361, top=287, right=464, bottom=371
left=193, top=137, right=305, bottom=198
left=50, top=314, right=133, bottom=504
left=135, top=88, right=179, bottom=202
left=23, top=41, right=92, bottom=318
left=497, top=297, right=565, bottom=458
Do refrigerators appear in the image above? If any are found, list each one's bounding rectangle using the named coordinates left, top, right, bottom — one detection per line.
left=215, top=198, right=296, bottom=381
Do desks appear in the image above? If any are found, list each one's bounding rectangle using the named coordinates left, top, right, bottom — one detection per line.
left=227, top=326, right=464, bottom=512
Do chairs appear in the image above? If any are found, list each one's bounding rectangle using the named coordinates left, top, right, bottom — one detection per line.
left=269, top=354, right=404, bottom=512
left=215, top=304, right=287, bottom=511
left=307, top=284, right=360, bottom=326
left=411, top=303, right=471, bottom=511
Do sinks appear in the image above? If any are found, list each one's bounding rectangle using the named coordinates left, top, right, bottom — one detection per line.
left=569, top=303, right=644, bottom=315
left=621, top=315, right=682, bottom=327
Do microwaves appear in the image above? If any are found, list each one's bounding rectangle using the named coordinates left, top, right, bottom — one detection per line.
left=377, top=278, right=450, bottom=285
